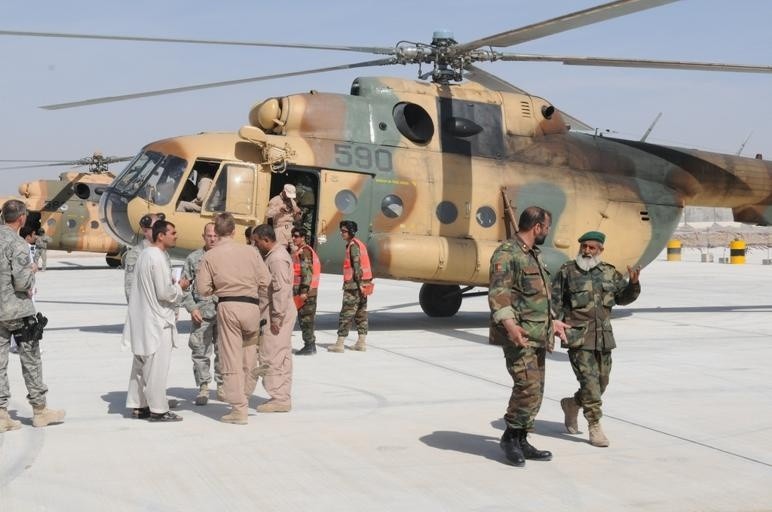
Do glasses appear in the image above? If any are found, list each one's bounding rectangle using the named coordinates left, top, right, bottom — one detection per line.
left=342, top=229, right=349, bottom=233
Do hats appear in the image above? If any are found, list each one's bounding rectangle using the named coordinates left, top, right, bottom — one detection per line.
left=579, top=231, right=606, bottom=244
left=284, top=184, right=297, bottom=199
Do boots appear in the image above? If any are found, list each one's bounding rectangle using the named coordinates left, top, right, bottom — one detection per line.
left=520, top=431, right=551, bottom=460
left=32, top=404, right=65, bottom=427
left=589, top=419, right=609, bottom=447
left=328, top=336, right=345, bottom=353
left=217, top=384, right=227, bottom=401
left=500, top=428, right=524, bottom=466
left=349, top=335, right=366, bottom=352
left=561, top=397, right=582, bottom=433
left=195, top=384, right=209, bottom=406
left=0, top=408, right=21, bottom=434
left=295, top=341, right=316, bottom=355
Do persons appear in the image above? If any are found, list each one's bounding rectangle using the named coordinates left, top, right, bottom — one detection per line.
left=328, top=220, right=373, bottom=353
left=180, top=213, right=297, bottom=424
left=176, top=173, right=223, bottom=211
left=19, top=227, right=36, bottom=244
left=550, top=231, right=641, bottom=448
left=0, top=199, right=65, bottom=433
left=266, top=173, right=320, bottom=354
left=33, top=228, right=52, bottom=272
left=125, top=213, right=190, bottom=421
left=488, top=207, right=572, bottom=467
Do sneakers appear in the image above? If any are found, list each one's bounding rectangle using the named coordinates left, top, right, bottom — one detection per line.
left=149, top=412, right=183, bottom=422
left=221, top=411, right=247, bottom=425
left=256, top=403, right=290, bottom=411
left=132, top=406, right=148, bottom=419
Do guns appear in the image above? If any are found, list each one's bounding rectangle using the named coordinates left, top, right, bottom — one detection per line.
left=31, top=313, right=48, bottom=351
